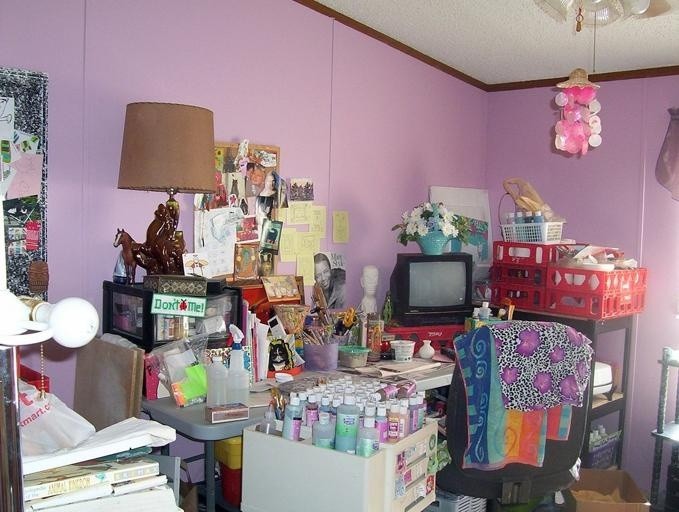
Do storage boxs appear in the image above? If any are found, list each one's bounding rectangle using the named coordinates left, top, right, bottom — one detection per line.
left=214, top=438, right=242, bottom=470
left=219, top=460, right=242, bottom=507
left=562, top=469, right=651, bottom=512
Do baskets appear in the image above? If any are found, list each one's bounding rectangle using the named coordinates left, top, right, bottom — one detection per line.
left=583, top=434, right=619, bottom=472
left=499, top=221, right=570, bottom=247
left=488, top=239, right=650, bottom=321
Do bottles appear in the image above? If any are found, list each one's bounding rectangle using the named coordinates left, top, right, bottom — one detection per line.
left=208, top=354, right=225, bottom=406
left=590, top=423, right=608, bottom=445
left=419, top=340, right=433, bottom=358
left=262, top=376, right=428, bottom=456
left=269, top=344, right=289, bottom=371
left=505, top=211, right=545, bottom=242
left=472, top=301, right=506, bottom=321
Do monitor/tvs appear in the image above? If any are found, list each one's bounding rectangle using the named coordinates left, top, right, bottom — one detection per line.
left=389, top=252, right=472, bottom=326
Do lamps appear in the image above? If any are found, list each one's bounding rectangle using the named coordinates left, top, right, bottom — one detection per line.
left=532, top=0, right=651, bottom=26
left=17, top=291, right=100, bottom=349
left=117, top=100, right=217, bottom=298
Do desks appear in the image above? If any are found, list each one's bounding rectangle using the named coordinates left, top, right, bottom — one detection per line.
left=140, top=349, right=457, bottom=512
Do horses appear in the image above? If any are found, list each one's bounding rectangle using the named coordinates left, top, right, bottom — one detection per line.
left=113, top=228, right=146, bottom=286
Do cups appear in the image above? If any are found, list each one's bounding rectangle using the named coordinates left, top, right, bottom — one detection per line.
left=389, top=340, right=415, bottom=362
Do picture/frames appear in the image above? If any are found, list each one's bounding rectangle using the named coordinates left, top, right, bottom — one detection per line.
left=195, top=143, right=280, bottom=285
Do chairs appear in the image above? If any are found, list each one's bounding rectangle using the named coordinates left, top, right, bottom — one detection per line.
left=436, top=320, right=597, bottom=512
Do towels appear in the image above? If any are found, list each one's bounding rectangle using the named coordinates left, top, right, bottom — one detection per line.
left=452, top=325, right=571, bottom=471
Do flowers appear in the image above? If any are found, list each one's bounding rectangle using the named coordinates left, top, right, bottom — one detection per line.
left=391, top=201, right=468, bottom=246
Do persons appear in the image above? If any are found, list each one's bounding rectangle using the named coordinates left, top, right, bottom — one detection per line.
left=262, top=171, right=276, bottom=194
left=252, top=169, right=265, bottom=193
left=313, top=253, right=346, bottom=309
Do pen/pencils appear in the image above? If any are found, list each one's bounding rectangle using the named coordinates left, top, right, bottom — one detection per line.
left=301, top=327, right=335, bottom=345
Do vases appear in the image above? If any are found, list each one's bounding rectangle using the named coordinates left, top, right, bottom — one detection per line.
left=414, top=202, right=454, bottom=256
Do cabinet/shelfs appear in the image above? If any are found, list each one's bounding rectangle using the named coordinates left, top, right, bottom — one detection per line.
left=649, top=347, right=679, bottom=512
left=471, top=303, right=633, bottom=469
left=239, top=417, right=438, bottom=512
left=102, top=278, right=243, bottom=397
